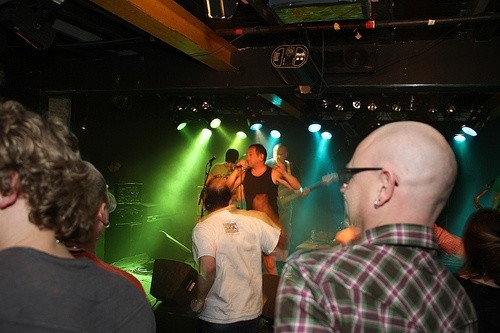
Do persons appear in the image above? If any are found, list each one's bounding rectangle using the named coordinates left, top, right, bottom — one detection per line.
left=190, top=121, right=500, bottom=333
left=0, top=100, right=156, bottom=333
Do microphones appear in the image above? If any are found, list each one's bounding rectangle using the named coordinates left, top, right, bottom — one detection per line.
left=206, top=156, right=216, bottom=164
left=239, top=154, right=246, bottom=159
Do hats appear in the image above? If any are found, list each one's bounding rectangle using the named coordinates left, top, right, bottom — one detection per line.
left=226, top=149, right=239, bottom=163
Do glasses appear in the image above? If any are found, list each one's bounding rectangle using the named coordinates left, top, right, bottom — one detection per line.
left=341, top=168, right=398, bottom=186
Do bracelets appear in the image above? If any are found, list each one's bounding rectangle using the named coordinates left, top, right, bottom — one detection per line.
left=192, top=298, right=203, bottom=307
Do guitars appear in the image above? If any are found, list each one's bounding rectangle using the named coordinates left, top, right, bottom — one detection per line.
left=276, top=173, right=339, bottom=210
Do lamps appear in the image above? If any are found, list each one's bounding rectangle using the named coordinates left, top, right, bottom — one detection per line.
left=405, top=94, right=416, bottom=111
left=461, top=92, right=500, bottom=136
left=168, top=95, right=221, bottom=131
left=244, top=105, right=264, bottom=131
left=302, top=100, right=321, bottom=133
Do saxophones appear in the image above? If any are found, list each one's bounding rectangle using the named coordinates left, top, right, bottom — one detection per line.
left=473, top=180, right=494, bottom=209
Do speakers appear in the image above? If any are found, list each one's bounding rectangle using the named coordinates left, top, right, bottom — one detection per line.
left=150, top=259, right=199, bottom=306
left=261, top=274, right=281, bottom=323
